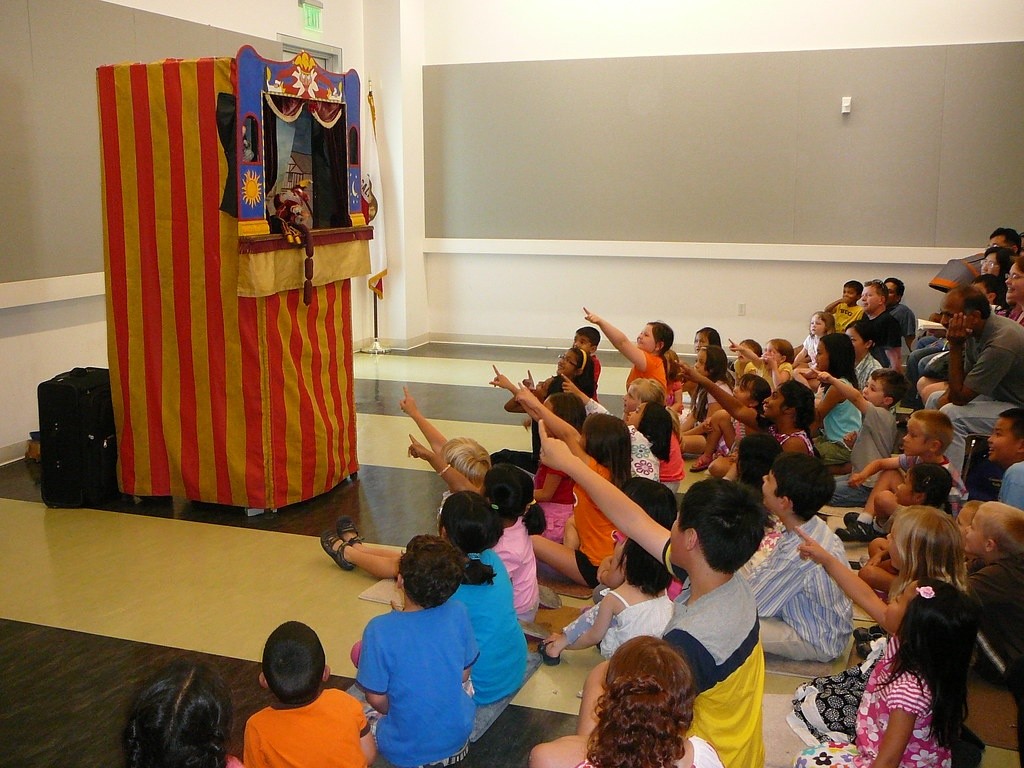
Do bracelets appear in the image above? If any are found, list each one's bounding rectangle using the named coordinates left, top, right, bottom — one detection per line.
left=436, top=463, right=453, bottom=475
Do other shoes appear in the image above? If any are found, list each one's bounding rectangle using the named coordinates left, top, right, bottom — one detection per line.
left=336, top=516, right=365, bottom=546
left=857, top=642, right=872, bottom=658
left=835, top=521, right=877, bottom=542
left=844, top=512, right=889, bottom=539
left=853, top=626, right=883, bottom=640
left=321, top=529, right=356, bottom=570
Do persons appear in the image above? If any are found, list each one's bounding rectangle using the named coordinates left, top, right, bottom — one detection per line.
left=397, top=224, right=1023, bottom=589
left=852, top=499, right=1024, bottom=689
left=352, top=533, right=479, bottom=768
left=573, top=635, right=727, bottom=768
left=792, top=523, right=983, bottom=767
left=525, top=418, right=768, bottom=767
left=350, top=489, right=529, bottom=707
left=858, top=461, right=953, bottom=601
left=783, top=505, right=987, bottom=767
left=119, top=656, right=249, bottom=768
left=404, top=434, right=563, bottom=642
left=320, top=437, right=493, bottom=578
left=745, top=451, right=855, bottom=664
left=244, top=620, right=378, bottom=768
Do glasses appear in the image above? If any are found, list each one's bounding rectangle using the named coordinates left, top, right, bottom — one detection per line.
left=558, top=354, right=577, bottom=366
left=875, top=280, right=884, bottom=290
left=981, top=260, right=999, bottom=269
left=1005, top=274, right=1024, bottom=279
left=988, top=245, right=1012, bottom=249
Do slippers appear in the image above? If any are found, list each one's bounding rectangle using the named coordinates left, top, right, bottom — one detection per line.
left=538, top=640, right=560, bottom=665
